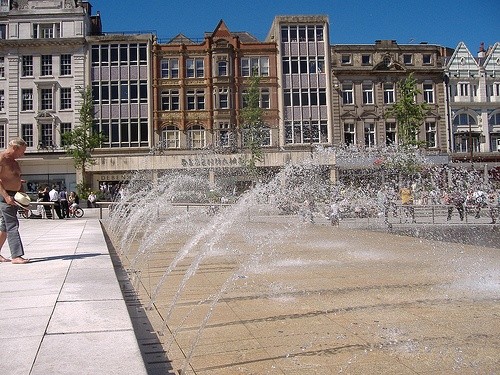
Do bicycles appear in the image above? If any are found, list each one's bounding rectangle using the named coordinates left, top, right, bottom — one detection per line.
left=68, top=207, right=84, bottom=218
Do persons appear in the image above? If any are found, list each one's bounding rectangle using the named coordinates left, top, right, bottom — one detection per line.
left=399, top=181, right=415, bottom=223
left=87, top=192, right=97, bottom=208
left=37, top=185, right=79, bottom=219
left=303, top=197, right=314, bottom=223
left=100, top=183, right=119, bottom=197
left=421, top=184, right=500, bottom=223
left=377, top=184, right=397, bottom=224
left=0, top=138, right=30, bottom=263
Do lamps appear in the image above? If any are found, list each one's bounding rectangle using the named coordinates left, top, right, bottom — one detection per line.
left=471, top=74, right=475, bottom=78
left=490, top=74, right=493, bottom=77
left=454, top=73, right=458, bottom=78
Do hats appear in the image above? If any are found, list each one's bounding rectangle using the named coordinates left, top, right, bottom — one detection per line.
left=13, top=191, right=31, bottom=210
